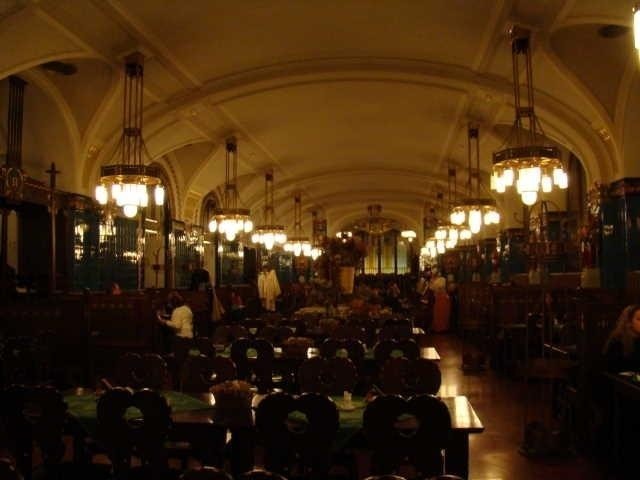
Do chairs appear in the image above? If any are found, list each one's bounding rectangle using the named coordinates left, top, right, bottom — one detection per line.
left=468, top=282, right=640, bottom=458
left=1, top=281, right=485, bottom=480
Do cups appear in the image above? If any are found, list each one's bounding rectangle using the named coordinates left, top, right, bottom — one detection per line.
left=343, top=391, right=352, bottom=407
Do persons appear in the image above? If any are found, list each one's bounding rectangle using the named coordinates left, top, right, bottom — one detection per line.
left=155, top=291, right=195, bottom=374
left=107, top=275, right=130, bottom=296
left=601, top=304, right=639, bottom=376
left=353, top=267, right=452, bottom=335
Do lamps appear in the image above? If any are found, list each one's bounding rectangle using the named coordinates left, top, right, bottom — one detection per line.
left=253, top=170, right=287, bottom=249
left=285, top=191, right=324, bottom=260
left=207, top=135, right=253, bottom=241
left=419, top=25, right=569, bottom=265
left=95, top=50, right=166, bottom=218
